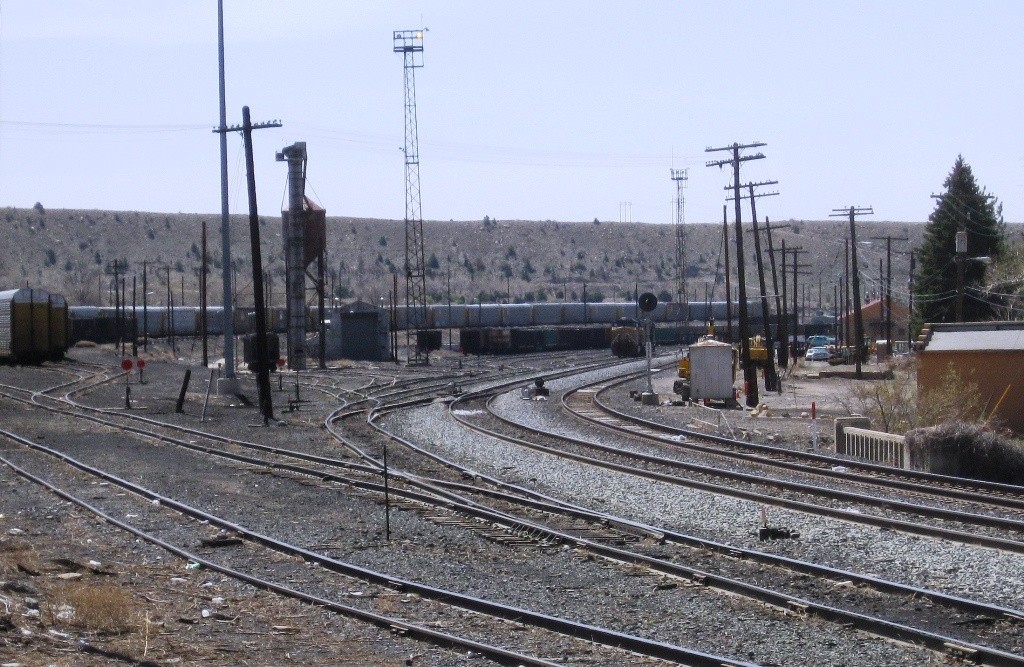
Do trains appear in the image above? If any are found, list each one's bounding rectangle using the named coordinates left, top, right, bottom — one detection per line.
left=0, top=287, right=779, bottom=365
